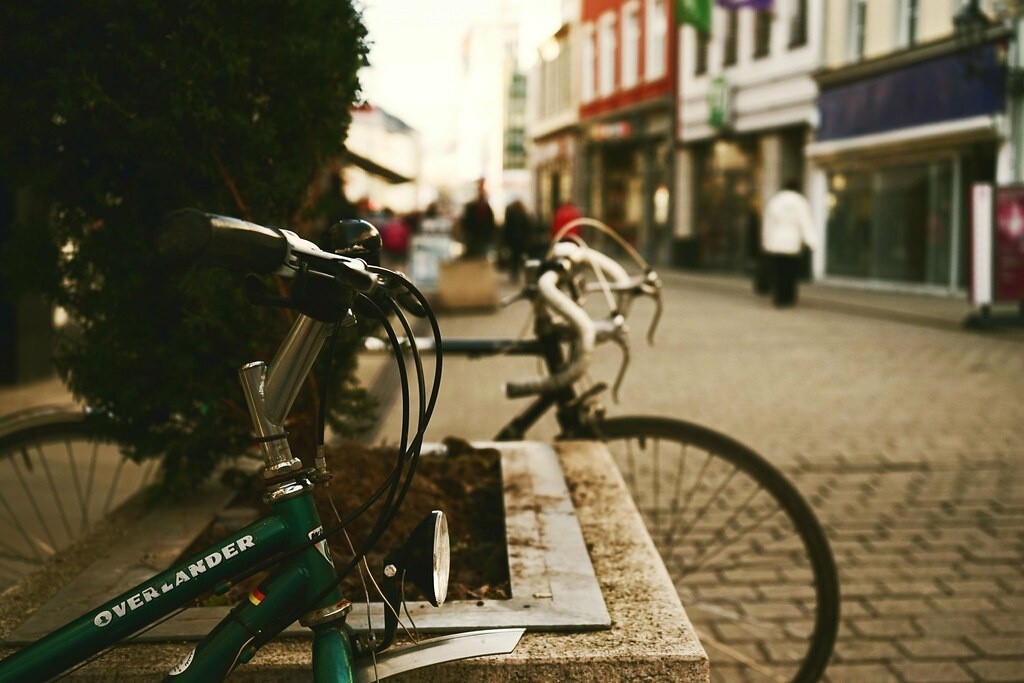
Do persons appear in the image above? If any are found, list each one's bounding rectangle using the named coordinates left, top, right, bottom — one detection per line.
left=759, top=179, right=817, bottom=305
left=495, top=202, right=538, bottom=281
left=549, top=185, right=581, bottom=241
left=459, top=178, right=496, bottom=259
left=357, top=184, right=456, bottom=255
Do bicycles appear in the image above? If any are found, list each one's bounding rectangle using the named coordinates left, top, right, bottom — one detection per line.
left=1, top=213, right=843, bottom=683
left=1, top=213, right=453, bottom=683
